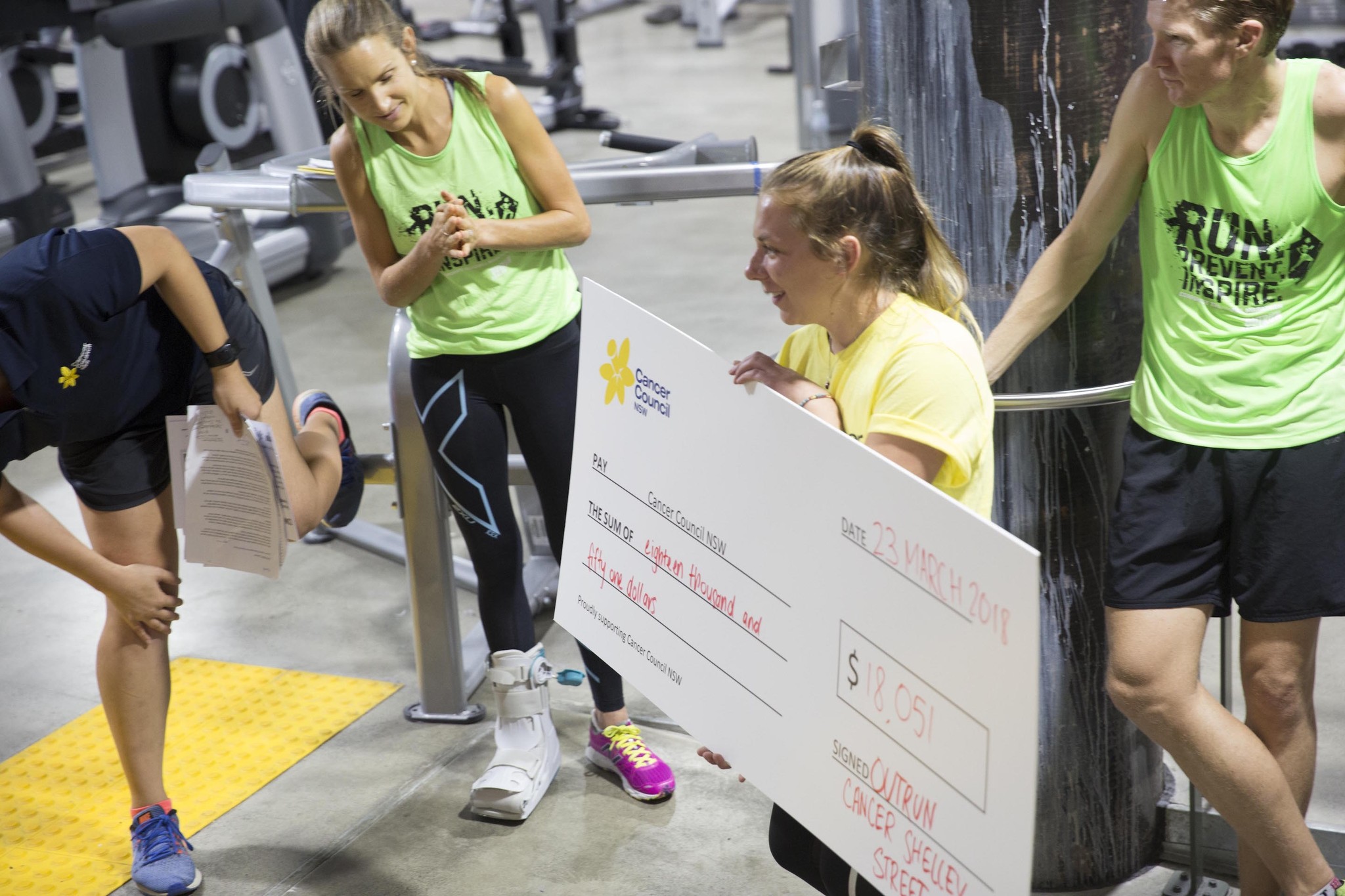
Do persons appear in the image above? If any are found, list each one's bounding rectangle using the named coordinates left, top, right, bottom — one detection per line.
left=304, top=2, right=679, bottom=825
left=978, top=0, right=1345, bottom=896
left=0, top=225, right=364, bottom=896
left=727, top=122, right=996, bottom=896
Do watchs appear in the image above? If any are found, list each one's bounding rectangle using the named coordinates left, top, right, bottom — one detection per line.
left=201, top=336, right=240, bottom=368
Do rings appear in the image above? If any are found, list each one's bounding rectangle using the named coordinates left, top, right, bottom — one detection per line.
left=441, top=229, right=449, bottom=235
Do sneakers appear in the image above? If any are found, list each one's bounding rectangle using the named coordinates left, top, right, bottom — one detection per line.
left=128, top=803, right=202, bottom=896
left=293, top=389, right=363, bottom=528
left=585, top=713, right=676, bottom=800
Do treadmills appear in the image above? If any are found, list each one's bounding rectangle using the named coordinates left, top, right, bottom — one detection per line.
left=0, top=1, right=355, bottom=297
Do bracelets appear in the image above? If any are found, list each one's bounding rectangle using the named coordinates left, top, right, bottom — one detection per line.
left=800, top=394, right=835, bottom=407
